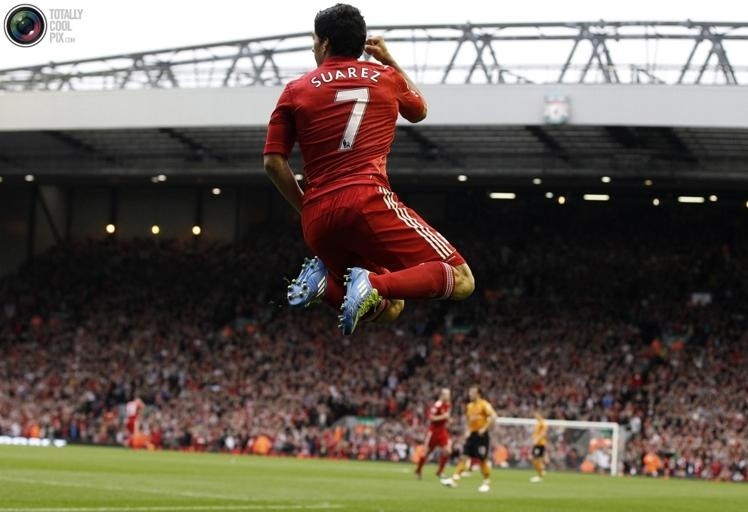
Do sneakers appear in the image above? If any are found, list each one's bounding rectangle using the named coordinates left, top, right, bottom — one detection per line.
left=285, top=255, right=330, bottom=308
left=335, top=265, right=384, bottom=337
left=476, top=479, right=490, bottom=493
left=437, top=477, right=458, bottom=488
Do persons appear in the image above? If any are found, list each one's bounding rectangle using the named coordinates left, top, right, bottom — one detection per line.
left=0, top=233, right=748, bottom=482
left=441, top=385, right=496, bottom=491
left=263, top=2, right=477, bottom=336
